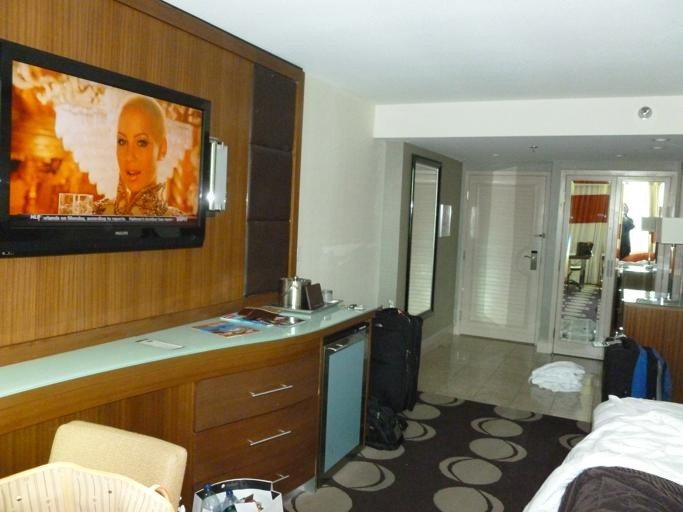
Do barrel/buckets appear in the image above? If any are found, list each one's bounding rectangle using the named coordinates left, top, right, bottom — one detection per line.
left=279, top=277, right=311, bottom=309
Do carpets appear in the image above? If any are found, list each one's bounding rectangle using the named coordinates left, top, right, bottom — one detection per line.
left=284, top=389, right=590, bottom=512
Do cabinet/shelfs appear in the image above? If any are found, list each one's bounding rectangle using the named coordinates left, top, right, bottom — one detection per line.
left=0, top=316, right=371, bottom=510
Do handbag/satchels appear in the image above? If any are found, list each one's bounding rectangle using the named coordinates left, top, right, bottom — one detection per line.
left=366, top=404, right=408, bottom=452
left=191, top=478, right=284, bottom=512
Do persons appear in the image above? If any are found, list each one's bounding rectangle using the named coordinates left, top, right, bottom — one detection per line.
left=619, top=201, right=635, bottom=259
left=58, top=96, right=185, bottom=218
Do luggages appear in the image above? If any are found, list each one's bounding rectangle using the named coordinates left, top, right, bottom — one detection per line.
left=601, top=333, right=673, bottom=401
left=368, top=307, right=422, bottom=413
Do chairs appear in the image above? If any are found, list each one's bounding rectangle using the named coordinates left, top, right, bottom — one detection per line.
left=47, top=420, right=187, bottom=510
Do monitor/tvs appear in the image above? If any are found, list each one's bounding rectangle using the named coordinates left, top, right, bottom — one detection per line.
left=0, top=38, right=211, bottom=259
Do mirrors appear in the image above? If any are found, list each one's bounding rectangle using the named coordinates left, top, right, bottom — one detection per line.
left=404, top=153, right=443, bottom=318
left=554, top=175, right=671, bottom=360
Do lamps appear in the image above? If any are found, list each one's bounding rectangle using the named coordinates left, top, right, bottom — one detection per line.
left=655, top=218, right=683, bottom=300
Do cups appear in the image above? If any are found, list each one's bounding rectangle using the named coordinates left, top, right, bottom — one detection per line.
left=322, top=290, right=333, bottom=303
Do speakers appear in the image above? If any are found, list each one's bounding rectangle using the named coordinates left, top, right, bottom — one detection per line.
left=209, top=142, right=229, bottom=212
